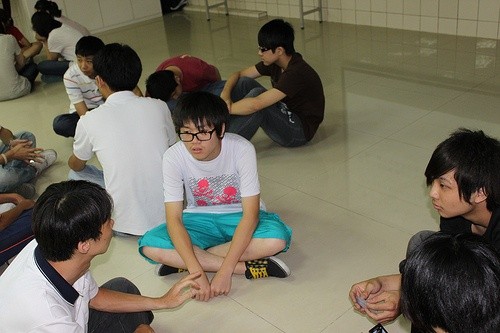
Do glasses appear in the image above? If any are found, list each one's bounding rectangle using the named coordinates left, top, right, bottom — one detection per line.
left=178, top=128, right=217, bottom=142
left=258, top=46, right=271, bottom=52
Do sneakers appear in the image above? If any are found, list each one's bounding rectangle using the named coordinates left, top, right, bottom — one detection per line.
left=159, top=264, right=187, bottom=276
left=30, top=149, right=57, bottom=175
left=245, top=256, right=290, bottom=280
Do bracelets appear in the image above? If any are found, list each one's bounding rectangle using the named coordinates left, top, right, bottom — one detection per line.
left=0, top=153, right=8, bottom=166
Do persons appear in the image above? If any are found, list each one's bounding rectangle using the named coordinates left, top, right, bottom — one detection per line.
left=67, top=42, right=184, bottom=240
left=219, top=19, right=327, bottom=149
left=52, top=35, right=144, bottom=140
left=0, top=0, right=95, bottom=103
left=0, top=123, right=57, bottom=190
left=0, top=191, right=36, bottom=266
left=398, top=230, right=500, bottom=333
left=348, top=127, right=500, bottom=326
left=0, top=180, right=202, bottom=333
left=143, top=53, right=227, bottom=109
left=137, top=92, right=291, bottom=279
